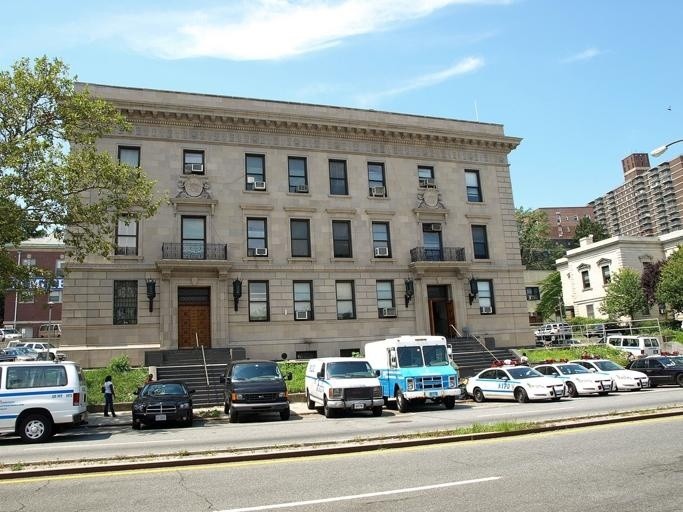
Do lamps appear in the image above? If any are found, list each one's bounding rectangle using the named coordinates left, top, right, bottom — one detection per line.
left=468, top=274, right=479, bottom=306
left=404, top=277, right=414, bottom=308
left=231, top=276, right=242, bottom=314
left=145, top=279, right=156, bottom=313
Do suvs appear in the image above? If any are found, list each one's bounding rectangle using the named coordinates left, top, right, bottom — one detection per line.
left=533, top=323, right=572, bottom=342
left=625, top=358, right=683, bottom=388
left=219, top=359, right=292, bottom=422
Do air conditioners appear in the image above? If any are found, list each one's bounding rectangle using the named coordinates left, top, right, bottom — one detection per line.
left=480, top=305, right=492, bottom=314
left=382, top=307, right=397, bottom=317
left=372, top=185, right=386, bottom=195
left=374, top=247, right=387, bottom=257
left=254, top=181, right=266, bottom=190
left=295, top=311, right=308, bottom=319
left=297, top=183, right=308, bottom=192
left=192, top=163, right=203, bottom=172
left=254, top=247, right=267, bottom=256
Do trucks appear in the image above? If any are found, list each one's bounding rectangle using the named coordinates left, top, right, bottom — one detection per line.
left=364, top=335, right=461, bottom=413
left=0, top=361, right=87, bottom=443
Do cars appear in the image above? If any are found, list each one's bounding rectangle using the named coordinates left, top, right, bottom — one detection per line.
left=645, top=351, right=683, bottom=358
left=566, top=355, right=650, bottom=392
left=0, top=340, right=59, bottom=362
left=0, top=328, right=22, bottom=342
left=465, top=359, right=568, bottom=403
left=130, top=379, right=195, bottom=428
left=519, top=358, right=616, bottom=397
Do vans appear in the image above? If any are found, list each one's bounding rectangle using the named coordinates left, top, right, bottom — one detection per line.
left=595, top=335, right=661, bottom=359
left=38, top=324, right=61, bottom=338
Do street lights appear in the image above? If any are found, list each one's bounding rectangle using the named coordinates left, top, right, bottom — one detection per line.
left=46, top=301, right=54, bottom=361
left=650, top=139, right=682, bottom=158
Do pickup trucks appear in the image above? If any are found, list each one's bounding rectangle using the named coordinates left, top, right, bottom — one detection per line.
left=304, top=357, right=384, bottom=417
left=583, top=322, right=640, bottom=338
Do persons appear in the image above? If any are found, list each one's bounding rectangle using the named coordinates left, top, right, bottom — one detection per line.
left=102, top=374, right=117, bottom=418
left=145, top=373, right=156, bottom=385
left=521, top=353, right=529, bottom=364
